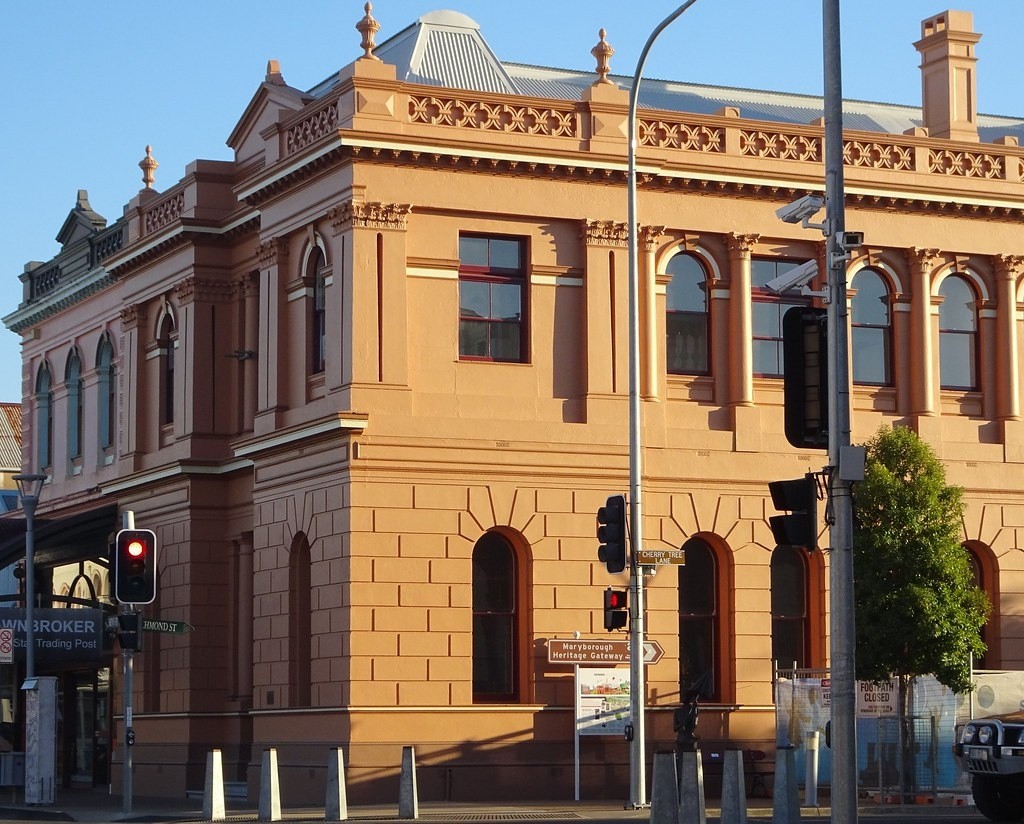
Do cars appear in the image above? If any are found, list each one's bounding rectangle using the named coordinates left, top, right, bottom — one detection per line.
left=954, top=703, right=1024, bottom=822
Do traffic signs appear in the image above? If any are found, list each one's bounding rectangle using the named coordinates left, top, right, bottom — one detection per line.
left=548, top=638, right=665, bottom=665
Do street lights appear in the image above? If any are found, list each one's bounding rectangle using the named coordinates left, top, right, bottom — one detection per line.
left=11, top=474, right=57, bottom=807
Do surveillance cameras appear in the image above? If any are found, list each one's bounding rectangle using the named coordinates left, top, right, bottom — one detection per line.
left=836, top=231, right=864, bottom=250
left=775, top=193, right=824, bottom=224
left=764, top=258, right=819, bottom=295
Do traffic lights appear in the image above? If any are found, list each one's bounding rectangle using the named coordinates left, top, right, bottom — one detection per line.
left=596, top=494, right=626, bottom=573
left=604, top=588, right=629, bottom=631
left=115, top=529, right=158, bottom=606
left=117, top=613, right=143, bottom=654
left=769, top=475, right=819, bottom=553
